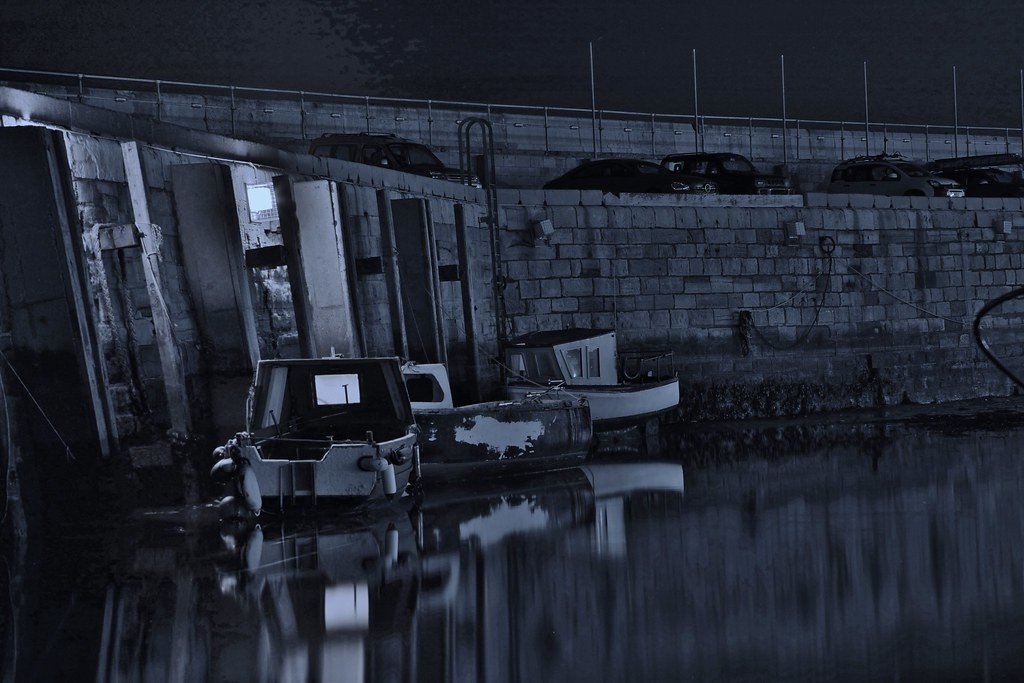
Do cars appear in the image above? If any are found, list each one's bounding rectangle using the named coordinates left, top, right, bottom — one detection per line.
left=543, top=160, right=720, bottom=195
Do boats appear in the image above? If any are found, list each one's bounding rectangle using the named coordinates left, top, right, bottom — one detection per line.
left=210, top=355, right=422, bottom=524
left=397, top=361, right=595, bottom=485
left=506, top=327, right=681, bottom=433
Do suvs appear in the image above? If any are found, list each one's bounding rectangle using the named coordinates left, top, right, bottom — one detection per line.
left=660, top=152, right=797, bottom=195
left=307, top=132, right=483, bottom=190
left=830, top=154, right=967, bottom=197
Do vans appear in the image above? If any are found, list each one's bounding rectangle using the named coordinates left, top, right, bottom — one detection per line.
left=930, top=154, right=1024, bottom=197
